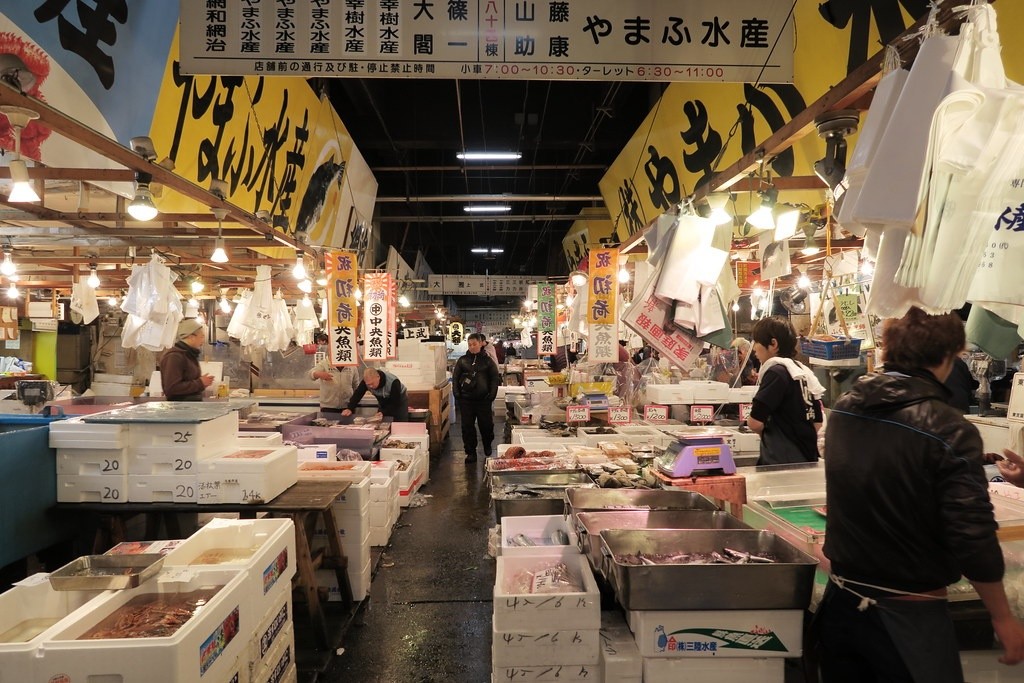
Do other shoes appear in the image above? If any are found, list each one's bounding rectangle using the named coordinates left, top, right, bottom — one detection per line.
left=465, top=452, right=477, bottom=463
left=484, top=446, right=492, bottom=456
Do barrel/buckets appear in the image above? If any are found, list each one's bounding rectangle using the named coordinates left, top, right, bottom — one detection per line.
left=961, top=304, right=1023, bottom=361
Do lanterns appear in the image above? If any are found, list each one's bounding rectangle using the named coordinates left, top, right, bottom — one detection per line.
left=449, top=321, right=463, bottom=346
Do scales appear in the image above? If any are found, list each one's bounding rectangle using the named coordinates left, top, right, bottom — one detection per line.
left=577, top=378, right=616, bottom=411
left=653, top=425, right=738, bottom=477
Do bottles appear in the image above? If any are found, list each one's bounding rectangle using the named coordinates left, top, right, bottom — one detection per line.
left=18, top=358, right=24, bottom=370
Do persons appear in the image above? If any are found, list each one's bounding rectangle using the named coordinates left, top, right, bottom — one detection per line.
left=747, top=316, right=827, bottom=471
left=317, top=334, right=327, bottom=345
left=506, top=343, right=516, bottom=358
left=944, top=357, right=979, bottom=414
left=452, top=333, right=500, bottom=463
left=712, top=337, right=760, bottom=413
left=341, top=367, right=408, bottom=422
left=480, top=334, right=506, bottom=385
left=308, top=358, right=360, bottom=414
left=159, top=318, right=215, bottom=402
left=809, top=306, right=1024, bottom=683
left=550, top=336, right=659, bottom=413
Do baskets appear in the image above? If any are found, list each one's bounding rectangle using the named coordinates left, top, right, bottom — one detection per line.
left=801, top=334, right=865, bottom=360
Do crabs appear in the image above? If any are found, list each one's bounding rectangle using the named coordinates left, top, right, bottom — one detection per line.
left=84, top=592, right=218, bottom=639
left=383, top=439, right=416, bottom=449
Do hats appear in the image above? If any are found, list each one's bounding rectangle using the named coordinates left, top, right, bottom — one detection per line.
left=177, top=317, right=203, bottom=339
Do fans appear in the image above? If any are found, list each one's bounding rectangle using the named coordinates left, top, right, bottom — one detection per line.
left=779, top=283, right=807, bottom=315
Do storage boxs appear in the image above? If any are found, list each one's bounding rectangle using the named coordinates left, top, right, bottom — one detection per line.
left=495, top=336, right=1024, bottom=683
left=0, top=338, right=454, bottom=683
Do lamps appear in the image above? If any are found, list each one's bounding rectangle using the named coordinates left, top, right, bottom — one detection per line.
left=218, top=288, right=232, bottom=313
left=813, top=109, right=860, bottom=200
left=0, top=245, right=15, bottom=275
left=0, top=105, right=42, bottom=203
left=297, top=272, right=316, bottom=293
left=569, top=269, right=590, bottom=286
left=705, top=193, right=731, bottom=224
left=618, top=253, right=629, bottom=284
left=88, top=253, right=100, bottom=287
left=209, top=208, right=231, bottom=263
left=8, top=277, right=19, bottom=298
left=746, top=163, right=819, bottom=255
left=315, top=261, right=327, bottom=285
left=798, top=264, right=810, bottom=286
left=293, top=250, right=306, bottom=279
left=127, top=171, right=158, bottom=221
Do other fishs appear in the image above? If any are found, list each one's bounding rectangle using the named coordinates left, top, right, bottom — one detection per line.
left=507, top=534, right=537, bottom=546
left=490, top=481, right=596, bottom=499
left=552, top=561, right=584, bottom=592
left=585, top=463, right=648, bottom=489
left=491, top=446, right=566, bottom=471
left=551, top=529, right=570, bottom=545
left=617, top=547, right=785, bottom=565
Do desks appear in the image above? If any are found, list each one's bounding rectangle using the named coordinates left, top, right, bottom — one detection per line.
left=55, top=479, right=354, bottom=646
